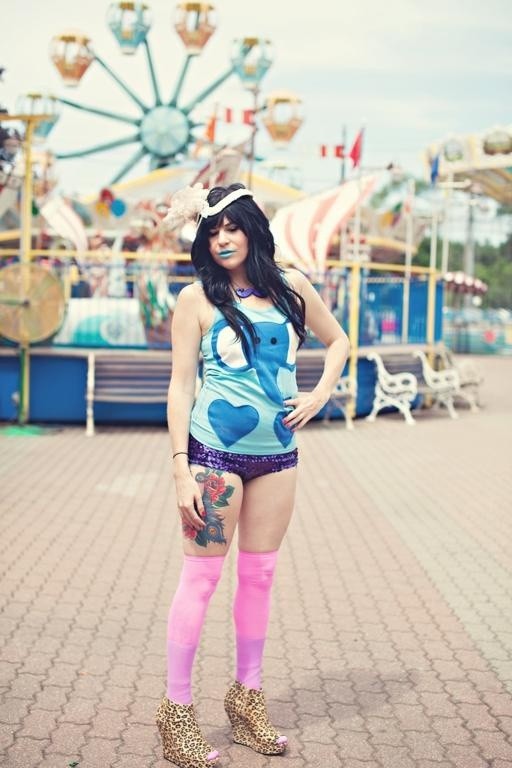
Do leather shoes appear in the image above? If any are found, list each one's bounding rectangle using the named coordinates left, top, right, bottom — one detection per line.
left=223, top=680, right=288, bottom=755
left=156, top=697, right=221, bottom=768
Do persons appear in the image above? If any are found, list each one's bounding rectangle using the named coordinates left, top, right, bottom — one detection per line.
left=155, top=179, right=354, bottom=768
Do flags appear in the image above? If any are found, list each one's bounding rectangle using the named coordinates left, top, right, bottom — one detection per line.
left=429, top=150, right=443, bottom=184
left=347, top=129, right=363, bottom=170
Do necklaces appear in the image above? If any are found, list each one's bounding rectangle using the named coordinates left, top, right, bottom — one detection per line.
left=229, top=276, right=268, bottom=299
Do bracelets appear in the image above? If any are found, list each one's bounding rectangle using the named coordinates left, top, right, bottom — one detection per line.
left=172, top=451, right=188, bottom=461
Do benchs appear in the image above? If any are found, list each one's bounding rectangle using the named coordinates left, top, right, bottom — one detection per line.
left=84, top=350, right=358, bottom=435
left=366, top=342, right=484, bottom=425
